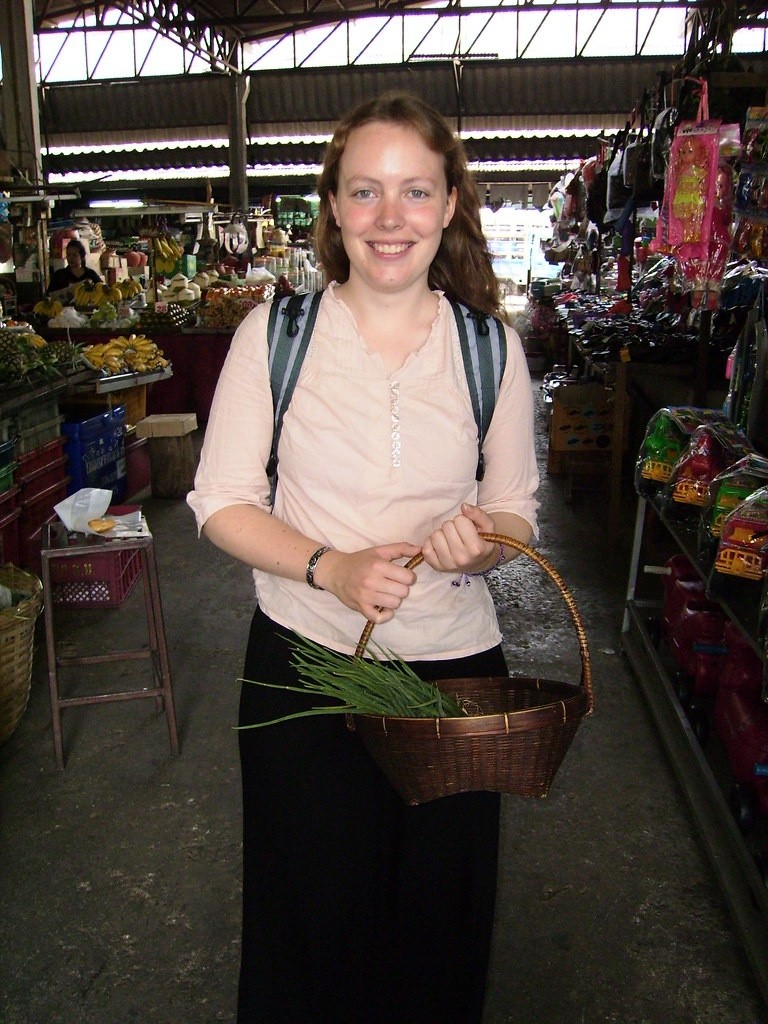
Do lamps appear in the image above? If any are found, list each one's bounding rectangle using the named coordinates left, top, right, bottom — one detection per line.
left=181, top=212, right=203, bottom=223
left=221, top=208, right=249, bottom=233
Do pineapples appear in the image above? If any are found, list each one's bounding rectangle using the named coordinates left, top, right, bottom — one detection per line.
left=0, top=330, right=78, bottom=378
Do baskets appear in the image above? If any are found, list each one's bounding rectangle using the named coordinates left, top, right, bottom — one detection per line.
left=345, top=533, right=593, bottom=808
left=0, top=563, right=44, bottom=741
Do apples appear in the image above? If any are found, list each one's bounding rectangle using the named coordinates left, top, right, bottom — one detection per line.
left=92, top=302, right=138, bottom=328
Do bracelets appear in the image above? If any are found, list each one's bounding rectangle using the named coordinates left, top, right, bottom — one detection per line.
left=451, top=541, right=505, bottom=587
left=306, top=546, right=332, bottom=591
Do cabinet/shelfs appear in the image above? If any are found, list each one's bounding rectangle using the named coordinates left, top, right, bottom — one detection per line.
left=620, top=408, right=768, bottom=1014
left=567, top=307, right=711, bottom=561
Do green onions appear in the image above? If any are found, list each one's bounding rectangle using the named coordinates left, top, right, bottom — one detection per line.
left=231, top=626, right=469, bottom=730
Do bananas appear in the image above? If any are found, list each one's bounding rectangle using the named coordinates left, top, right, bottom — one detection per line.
left=31, top=297, right=62, bottom=318
left=82, top=334, right=167, bottom=374
left=74, top=280, right=144, bottom=306
left=149, top=232, right=183, bottom=262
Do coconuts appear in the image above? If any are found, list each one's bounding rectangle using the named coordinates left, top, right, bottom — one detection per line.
left=145, top=269, right=219, bottom=302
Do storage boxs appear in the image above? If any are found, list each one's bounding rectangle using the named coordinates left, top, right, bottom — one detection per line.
left=540, top=386, right=631, bottom=452
left=153, top=255, right=197, bottom=279
left=16, top=238, right=151, bottom=282
left=0, top=381, right=198, bottom=609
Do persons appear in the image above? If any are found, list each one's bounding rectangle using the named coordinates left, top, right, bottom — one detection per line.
left=187, top=94, right=540, bottom=1023
left=46, top=241, right=102, bottom=296
